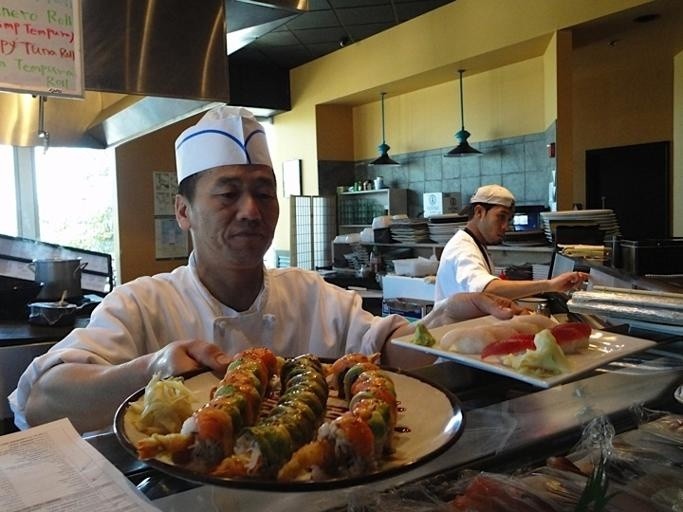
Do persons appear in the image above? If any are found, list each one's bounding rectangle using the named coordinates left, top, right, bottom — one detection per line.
left=5, top=103, right=529, bottom=435
left=432, top=183, right=591, bottom=312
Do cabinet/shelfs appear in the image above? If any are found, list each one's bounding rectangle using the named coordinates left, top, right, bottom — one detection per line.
left=333, top=176, right=591, bottom=313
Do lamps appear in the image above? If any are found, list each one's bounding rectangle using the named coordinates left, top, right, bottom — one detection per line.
left=369, top=71, right=483, bottom=167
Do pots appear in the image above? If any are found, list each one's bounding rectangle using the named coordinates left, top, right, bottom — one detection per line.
left=28, top=256, right=87, bottom=300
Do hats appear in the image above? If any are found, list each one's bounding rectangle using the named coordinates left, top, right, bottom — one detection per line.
left=174, top=105, right=274, bottom=186
left=458, top=182, right=515, bottom=217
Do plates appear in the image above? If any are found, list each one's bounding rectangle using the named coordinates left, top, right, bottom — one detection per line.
left=389, top=214, right=469, bottom=246
left=109, top=355, right=468, bottom=494
left=538, top=206, right=623, bottom=247
left=387, top=304, right=659, bottom=390
